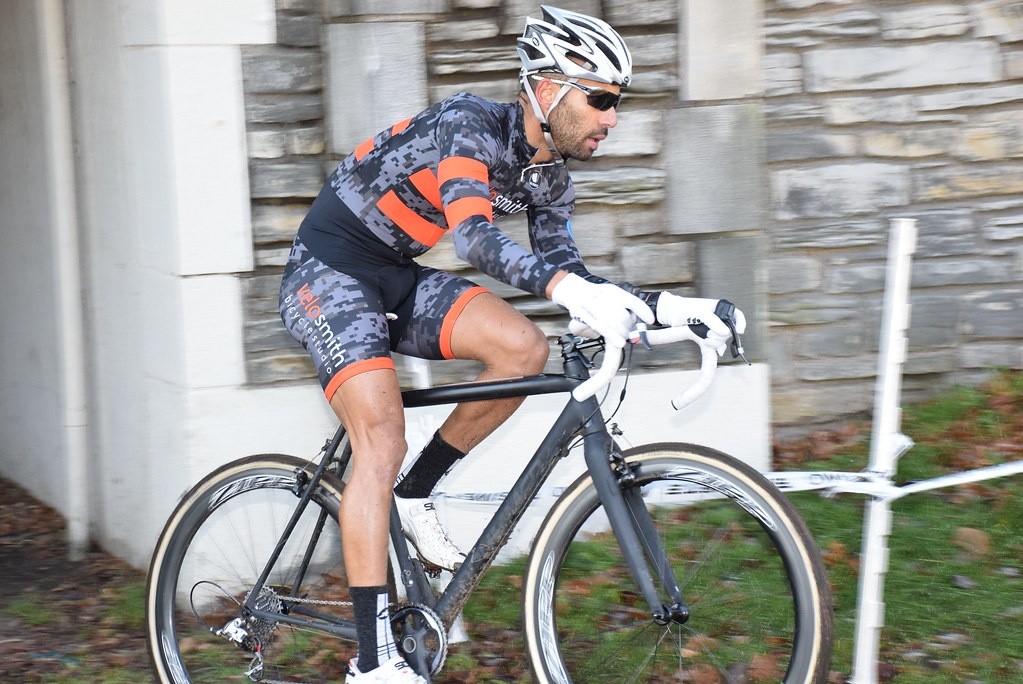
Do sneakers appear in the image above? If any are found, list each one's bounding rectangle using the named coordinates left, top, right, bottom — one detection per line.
left=392, top=489, right=467, bottom=572
left=344, top=656, right=428, bottom=684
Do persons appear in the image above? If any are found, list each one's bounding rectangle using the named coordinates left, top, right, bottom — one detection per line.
left=280, top=3, right=748, bottom=684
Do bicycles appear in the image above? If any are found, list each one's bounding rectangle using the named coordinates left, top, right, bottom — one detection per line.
left=145, top=299, right=833, bottom=684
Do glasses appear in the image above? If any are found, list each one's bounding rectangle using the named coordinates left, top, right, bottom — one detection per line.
left=531, top=75, right=621, bottom=112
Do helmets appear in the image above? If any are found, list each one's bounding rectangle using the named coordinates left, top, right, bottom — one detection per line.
left=516, top=5, right=633, bottom=87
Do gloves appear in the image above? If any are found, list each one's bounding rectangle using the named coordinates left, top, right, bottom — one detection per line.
left=656, top=291, right=747, bottom=357
left=552, top=272, right=655, bottom=349
